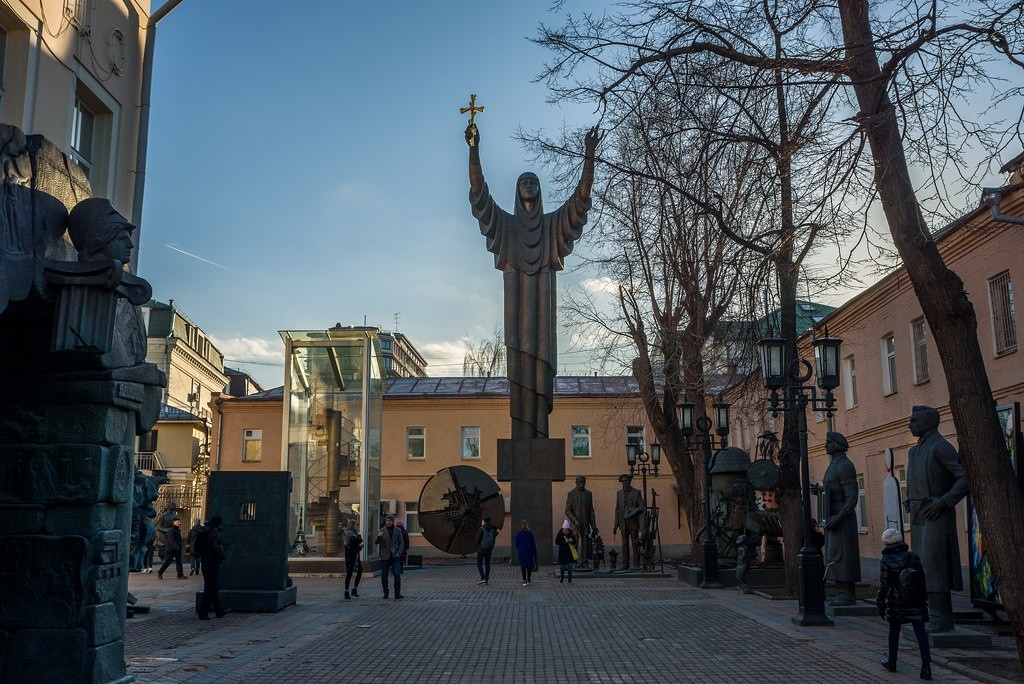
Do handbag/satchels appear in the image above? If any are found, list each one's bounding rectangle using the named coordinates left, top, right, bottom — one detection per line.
left=531, top=561, right=538, bottom=572
left=569, top=543, right=579, bottom=561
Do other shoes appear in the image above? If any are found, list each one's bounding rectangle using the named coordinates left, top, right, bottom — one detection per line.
left=523, top=580, right=530, bottom=586
left=478, top=579, right=488, bottom=585
left=568, top=581, right=573, bottom=585
left=559, top=578, right=563, bottom=582
left=177, top=576, right=188, bottom=580
left=143, top=568, right=153, bottom=574
left=190, top=568, right=199, bottom=575
left=199, top=613, right=210, bottom=620
left=218, top=607, right=232, bottom=618
left=383, top=594, right=389, bottom=599
left=157, top=571, right=162, bottom=579
left=395, top=593, right=404, bottom=599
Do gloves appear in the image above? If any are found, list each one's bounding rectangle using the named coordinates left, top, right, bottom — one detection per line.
left=878, top=604, right=887, bottom=621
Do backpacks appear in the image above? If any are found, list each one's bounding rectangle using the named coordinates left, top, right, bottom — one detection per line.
left=194, top=532, right=210, bottom=556
left=480, top=527, right=494, bottom=550
left=886, top=553, right=920, bottom=600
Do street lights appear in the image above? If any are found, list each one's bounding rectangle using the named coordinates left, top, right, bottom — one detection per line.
left=757, top=317, right=843, bottom=629
left=677, top=395, right=732, bottom=588
left=626, top=437, right=662, bottom=569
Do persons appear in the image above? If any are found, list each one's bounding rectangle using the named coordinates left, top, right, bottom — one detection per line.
left=812, top=436, right=862, bottom=606
left=344, top=519, right=364, bottom=599
left=735, top=535, right=753, bottom=594
left=555, top=520, right=577, bottom=583
left=198, top=516, right=232, bottom=619
left=901, top=404, right=972, bottom=633
left=187, top=519, right=203, bottom=576
left=157, top=502, right=177, bottom=543
left=613, top=475, right=646, bottom=571
left=391, top=520, right=409, bottom=574
left=566, top=475, right=598, bottom=568
left=158, top=518, right=188, bottom=580
left=465, top=122, right=605, bottom=438
left=143, top=543, right=154, bottom=573
left=876, top=528, right=932, bottom=680
left=375, top=514, right=404, bottom=598
left=515, top=520, right=537, bottom=586
left=475, top=516, right=499, bottom=585
left=68, top=198, right=147, bottom=372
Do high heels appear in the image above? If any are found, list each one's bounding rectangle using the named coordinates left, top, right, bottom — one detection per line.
left=344, top=591, right=351, bottom=599
left=351, top=588, right=358, bottom=597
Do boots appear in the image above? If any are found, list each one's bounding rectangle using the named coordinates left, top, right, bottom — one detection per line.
left=881, top=656, right=897, bottom=671
left=920, top=664, right=932, bottom=679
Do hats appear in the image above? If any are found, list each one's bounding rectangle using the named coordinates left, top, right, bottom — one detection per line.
left=882, top=529, right=903, bottom=545
left=482, top=516, right=491, bottom=523
left=396, top=522, right=402, bottom=526
left=562, top=519, right=571, bottom=529
